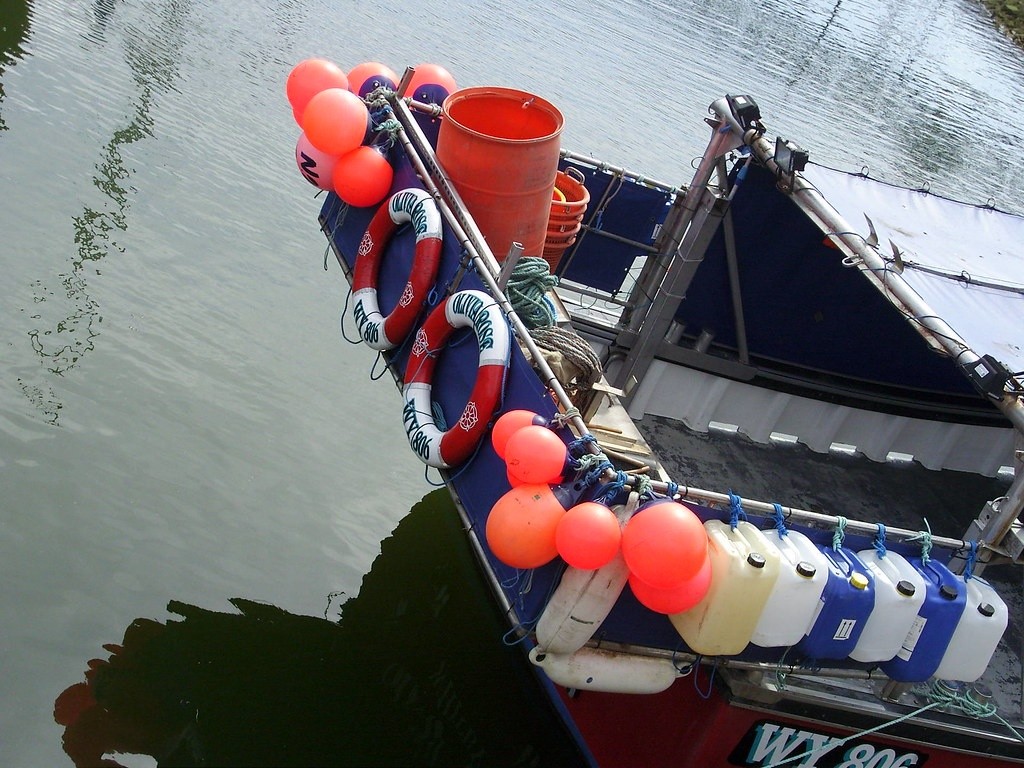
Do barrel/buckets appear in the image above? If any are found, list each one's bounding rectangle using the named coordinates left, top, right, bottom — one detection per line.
left=796, top=544, right=875, bottom=660
left=438, top=87, right=565, bottom=265
left=751, top=528, right=828, bottom=647
left=932, top=575, right=1008, bottom=682
left=848, top=549, right=927, bottom=662
left=667, top=520, right=781, bottom=656
left=876, top=558, right=967, bottom=682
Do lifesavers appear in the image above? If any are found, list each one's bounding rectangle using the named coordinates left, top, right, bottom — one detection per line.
left=348, top=185, right=446, bottom=355
left=400, top=286, right=515, bottom=473
